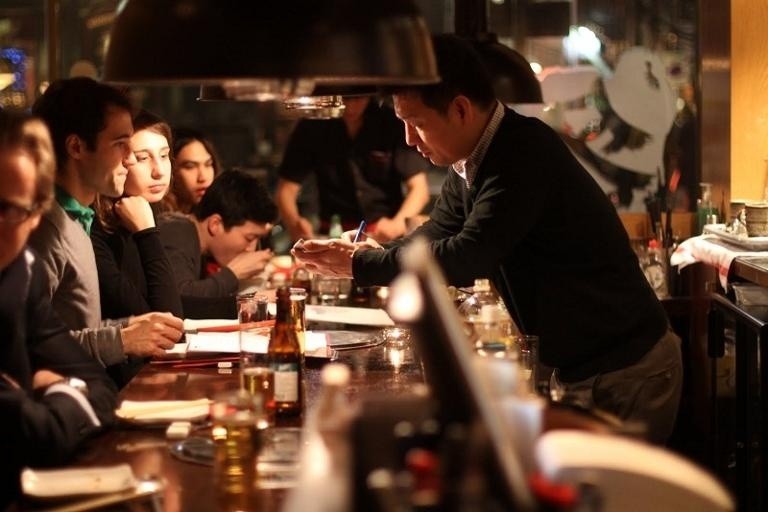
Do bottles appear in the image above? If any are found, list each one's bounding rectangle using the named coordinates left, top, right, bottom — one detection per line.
left=642, top=240, right=669, bottom=299
left=267, top=285, right=304, bottom=411
left=327, top=214, right=345, bottom=239
left=458, top=278, right=513, bottom=360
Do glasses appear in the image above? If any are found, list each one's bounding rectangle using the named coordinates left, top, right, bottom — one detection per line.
left=0, top=199, right=40, bottom=225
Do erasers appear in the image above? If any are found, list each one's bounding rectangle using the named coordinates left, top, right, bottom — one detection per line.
left=165, top=421, right=193, bottom=439
left=218, top=361, right=233, bottom=368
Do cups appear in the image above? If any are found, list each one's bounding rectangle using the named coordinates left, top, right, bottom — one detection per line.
left=236, top=295, right=268, bottom=323
left=318, top=276, right=350, bottom=301
left=730, top=201, right=768, bottom=238
left=517, top=336, right=542, bottom=388
left=206, top=360, right=274, bottom=475
left=287, top=289, right=308, bottom=333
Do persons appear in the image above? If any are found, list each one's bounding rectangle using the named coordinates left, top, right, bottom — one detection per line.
left=120, top=172, right=276, bottom=314
left=153, top=126, right=223, bottom=215
left=275, top=95, right=430, bottom=239
left=89, top=107, right=184, bottom=341
left=289, top=30, right=685, bottom=448
left=33, top=76, right=186, bottom=370
left=0, top=103, right=117, bottom=436
left=1, top=370, right=102, bottom=510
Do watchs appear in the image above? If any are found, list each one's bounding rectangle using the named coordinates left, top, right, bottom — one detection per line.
left=44, top=375, right=91, bottom=396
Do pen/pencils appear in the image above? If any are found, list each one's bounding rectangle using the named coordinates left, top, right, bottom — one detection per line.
left=353, top=220, right=366, bottom=243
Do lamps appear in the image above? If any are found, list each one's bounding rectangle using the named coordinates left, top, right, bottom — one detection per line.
left=98, top=1, right=440, bottom=105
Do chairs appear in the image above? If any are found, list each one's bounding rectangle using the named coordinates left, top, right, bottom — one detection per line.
left=706, top=291, right=768, bottom=511
